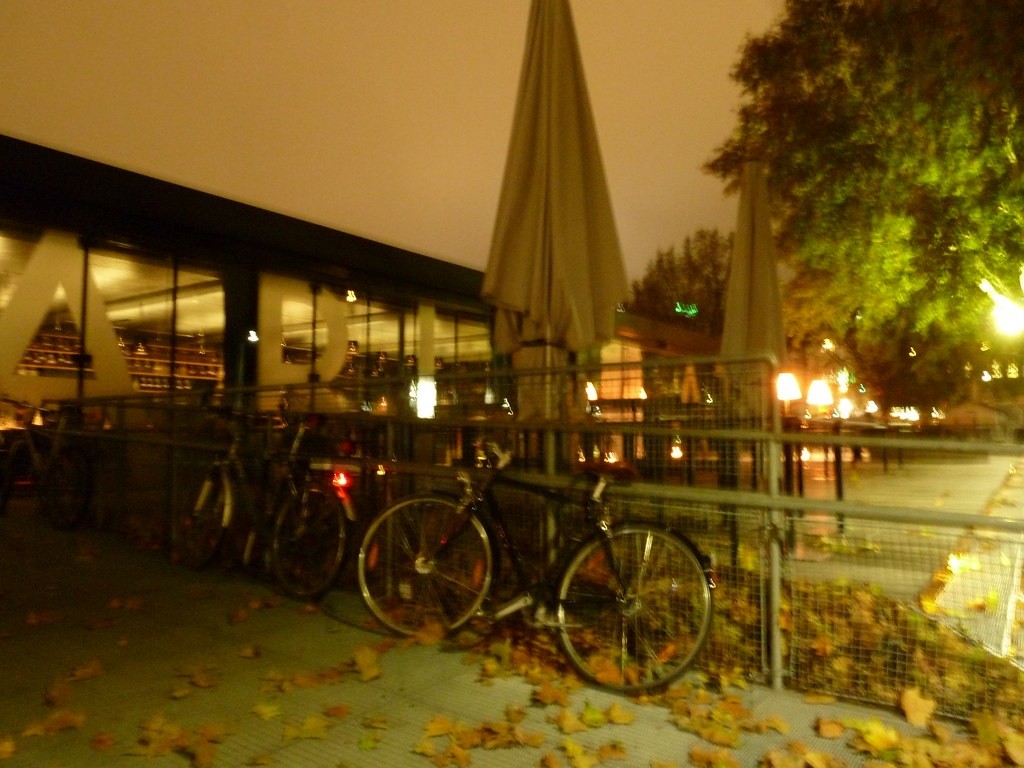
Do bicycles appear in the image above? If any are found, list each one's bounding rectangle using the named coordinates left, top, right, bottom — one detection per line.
left=176, top=400, right=357, bottom=601
left=355, top=426, right=718, bottom=698
left=0, top=394, right=97, bottom=531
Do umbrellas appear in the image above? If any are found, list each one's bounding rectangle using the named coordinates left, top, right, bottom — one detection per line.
left=477, top=1, right=633, bottom=362
left=715, top=149, right=788, bottom=383
